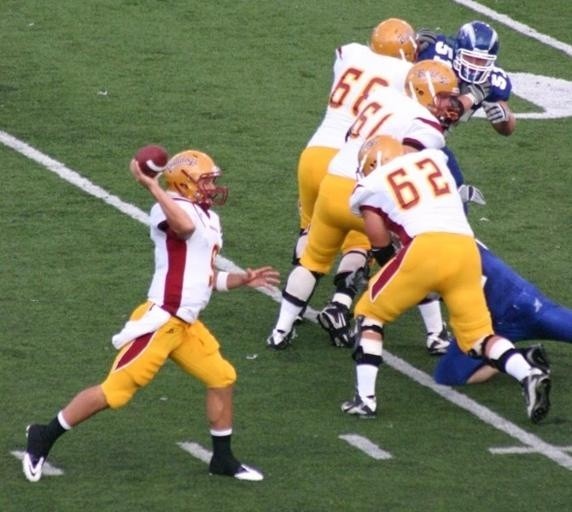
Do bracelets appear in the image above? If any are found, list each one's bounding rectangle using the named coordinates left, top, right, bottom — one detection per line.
left=215, top=270, right=230, bottom=294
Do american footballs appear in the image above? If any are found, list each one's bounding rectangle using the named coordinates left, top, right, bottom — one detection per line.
left=134, top=147, right=167, bottom=176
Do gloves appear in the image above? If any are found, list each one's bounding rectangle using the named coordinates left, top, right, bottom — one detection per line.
left=481, top=99, right=510, bottom=126
left=461, top=80, right=492, bottom=105
left=417, top=28, right=437, bottom=54
left=457, top=185, right=487, bottom=207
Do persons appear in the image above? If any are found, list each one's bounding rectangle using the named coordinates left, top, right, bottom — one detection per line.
left=19, top=140, right=281, bottom=484
left=414, top=19, right=518, bottom=218
left=289, top=16, right=435, bottom=354
left=429, top=233, right=570, bottom=388
left=262, top=57, right=490, bottom=354
left=337, top=133, right=553, bottom=427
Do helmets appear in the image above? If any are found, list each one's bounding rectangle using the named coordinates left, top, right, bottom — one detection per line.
left=162, top=149, right=229, bottom=208
left=404, top=60, right=460, bottom=125
left=355, top=134, right=407, bottom=183
left=452, top=20, right=499, bottom=84
left=368, top=16, right=420, bottom=64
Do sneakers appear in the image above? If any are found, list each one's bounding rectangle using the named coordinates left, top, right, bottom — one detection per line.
left=340, top=392, right=377, bottom=421
left=425, top=321, right=454, bottom=357
left=208, top=455, right=265, bottom=483
left=264, top=328, right=293, bottom=351
left=519, top=367, right=553, bottom=426
left=526, top=342, right=551, bottom=374
left=21, top=424, right=54, bottom=483
left=315, top=301, right=356, bottom=349
